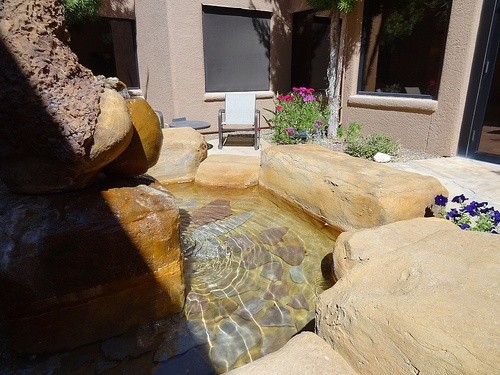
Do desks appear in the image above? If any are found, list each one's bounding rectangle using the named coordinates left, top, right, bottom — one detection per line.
left=168, top=119, right=211, bottom=131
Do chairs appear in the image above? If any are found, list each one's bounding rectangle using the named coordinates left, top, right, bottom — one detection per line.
left=217, top=93, right=262, bottom=150
